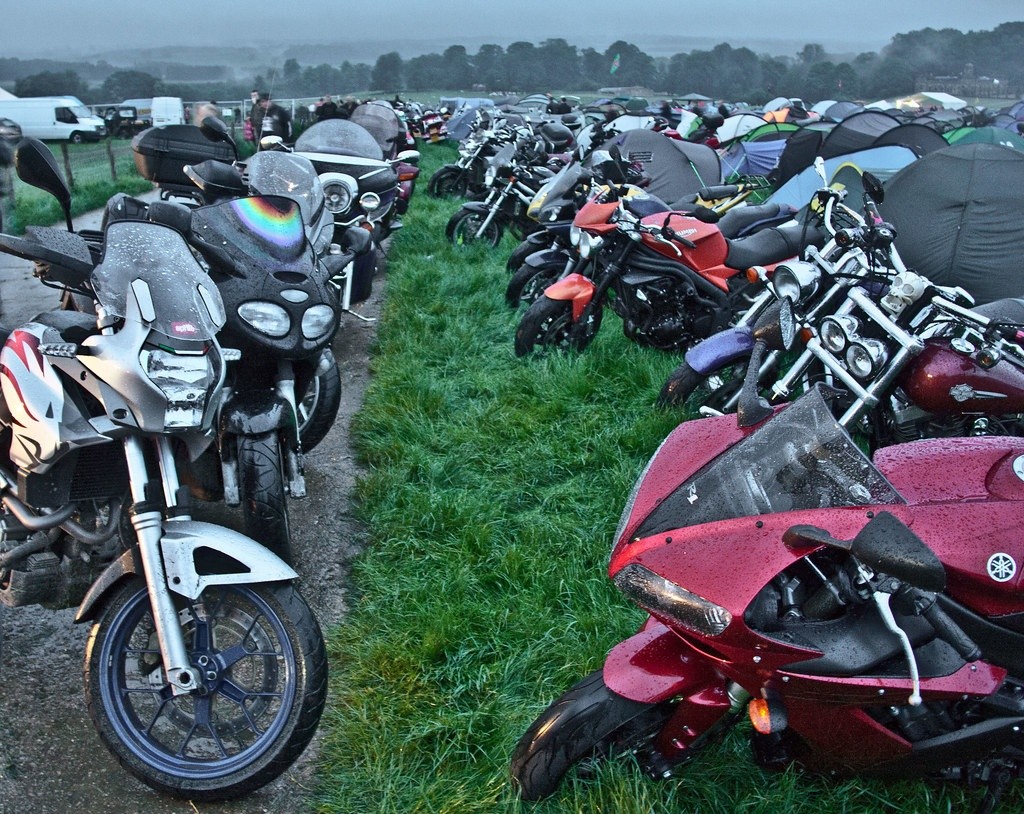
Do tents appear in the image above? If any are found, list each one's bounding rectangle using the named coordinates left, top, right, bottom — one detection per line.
left=406, top=88, right=1024, bottom=309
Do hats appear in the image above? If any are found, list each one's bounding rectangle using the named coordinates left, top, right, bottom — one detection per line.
left=256, top=94, right=272, bottom=105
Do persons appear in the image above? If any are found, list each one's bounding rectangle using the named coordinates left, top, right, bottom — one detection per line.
left=558, top=98, right=571, bottom=114
left=184, top=94, right=406, bottom=151
left=546, top=97, right=559, bottom=114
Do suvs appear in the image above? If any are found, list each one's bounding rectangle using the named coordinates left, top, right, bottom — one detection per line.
left=87, top=104, right=153, bottom=140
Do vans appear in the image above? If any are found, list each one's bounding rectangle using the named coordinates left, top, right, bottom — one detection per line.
left=0, top=96, right=107, bottom=144
left=106, top=97, right=186, bottom=128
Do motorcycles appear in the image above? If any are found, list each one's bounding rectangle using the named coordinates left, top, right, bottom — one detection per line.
left=658, top=155, right=1023, bottom=415
left=58, top=192, right=344, bottom=564
left=260, top=95, right=760, bottom=310
left=511, top=295, right=1023, bottom=814
left=696, top=172, right=1024, bottom=461
left=130, top=116, right=372, bottom=455
left=0, top=138, right=331, bottom=804
